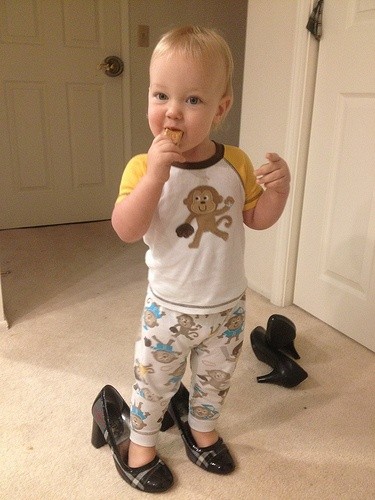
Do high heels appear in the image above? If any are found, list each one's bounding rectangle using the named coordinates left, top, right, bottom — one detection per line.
left=162, top=382, right=239, bottom=470
left=250, top=326, right=308, bottom=388
left=91, top=386, right=174, bottom=495
left=268, top=314, right=305, bottom=360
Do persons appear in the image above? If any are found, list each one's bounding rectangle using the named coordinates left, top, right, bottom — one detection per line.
left=111, top=26, right=291, bottom=470
